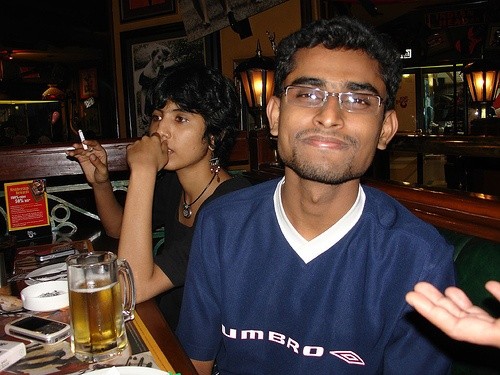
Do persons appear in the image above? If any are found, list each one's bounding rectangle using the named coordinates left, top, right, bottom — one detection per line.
left=66, top=65, right=255, bottom=324
left=139, top=46, right=170, bottom=131
left=176, top=18, right=456, bottom=375
left=406, top=280, right=500, bottom=349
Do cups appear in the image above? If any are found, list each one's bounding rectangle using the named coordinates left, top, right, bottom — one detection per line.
left=65, top=252, right=136, bottom=364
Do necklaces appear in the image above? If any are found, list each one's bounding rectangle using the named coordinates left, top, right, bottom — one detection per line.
left=182, top=168, right=221, bottom=218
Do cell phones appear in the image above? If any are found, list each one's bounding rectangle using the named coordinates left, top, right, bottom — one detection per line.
left=4, top=316, right=71, bottom=346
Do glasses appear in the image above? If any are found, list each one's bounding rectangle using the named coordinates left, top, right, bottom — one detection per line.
left=279, top=85, right=385, bottom=113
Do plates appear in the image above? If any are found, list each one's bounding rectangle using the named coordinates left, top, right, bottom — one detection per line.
left=25, top=262, right=68, bottom=285
left=81, top=366, right=169, bottom=375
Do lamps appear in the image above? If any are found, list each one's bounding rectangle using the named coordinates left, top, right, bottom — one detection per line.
left=233, top=39, right=278, bottom=133
left=463, top=46, right=500, bottom=119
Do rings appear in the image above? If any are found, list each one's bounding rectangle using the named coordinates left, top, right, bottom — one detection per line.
left=88, top=148, right=93, bottom=152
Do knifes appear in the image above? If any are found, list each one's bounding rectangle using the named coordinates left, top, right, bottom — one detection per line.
left=23, top=269, right=67, bottom=281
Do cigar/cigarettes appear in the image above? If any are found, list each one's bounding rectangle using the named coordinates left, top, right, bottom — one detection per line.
left=78, top=130, right=88, bottom=150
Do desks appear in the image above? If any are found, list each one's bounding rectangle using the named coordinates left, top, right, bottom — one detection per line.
left=0, top=236, right=197, bottom=375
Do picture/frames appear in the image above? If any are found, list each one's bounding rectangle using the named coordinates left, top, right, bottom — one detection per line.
left=119, top=21, right=221, bottom=137
left=118, top=0, right=177, bottom=24
